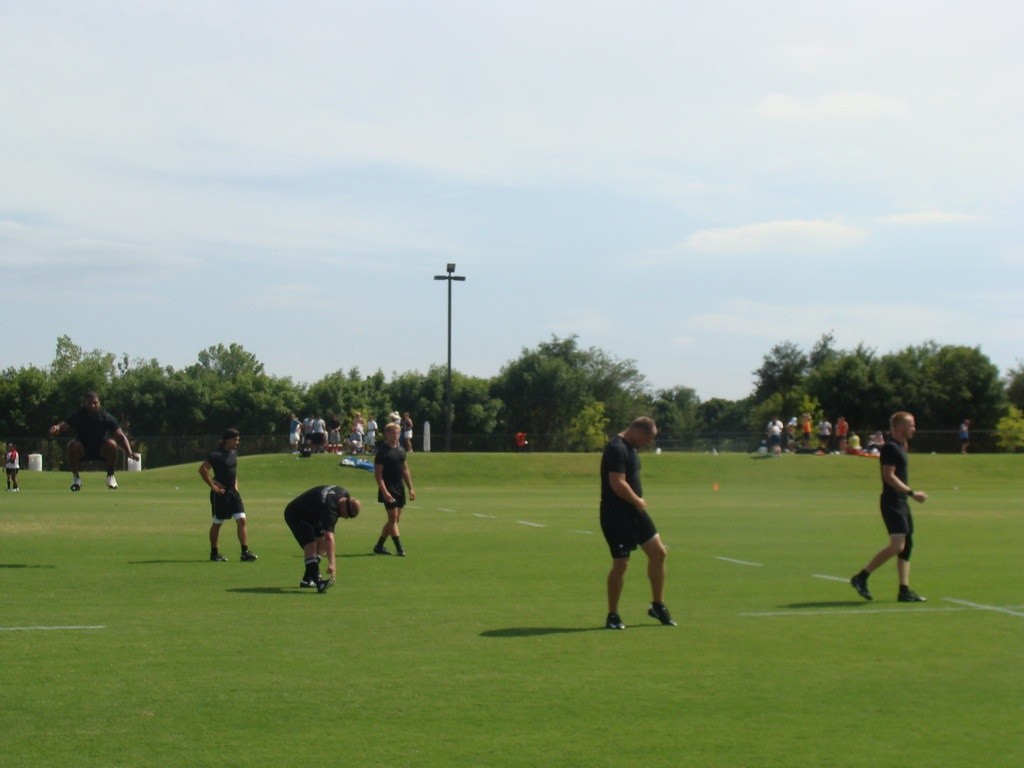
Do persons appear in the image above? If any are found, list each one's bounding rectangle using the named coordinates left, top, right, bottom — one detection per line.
left=600, top=415, right=678, bottom=630
left=284, top=484, right=361, bottom=594
left=199, top=428, right=258, bottom=562
left=2, top=442, right=20, bottom=492
left=47, top=390, right=140, bottom=492
left=289, top=411, right=413, bottom=458
left=514, top=431, right=529, bottom=453
left=760, top=412, right=885, bottom=455
left=959, top=420, right=970, bottom=455
left=374, top=422, right=416, bottom=557
left=851, top=412, right=928, bottom=603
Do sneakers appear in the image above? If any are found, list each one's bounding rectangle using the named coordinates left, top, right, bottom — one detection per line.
left=209, top=553, right=227, bottom=562
left=852, top=575, right=873, bottom=600
left=105, top=475, right=119, bottom=489
left=240, top=552, right=258, bottom=561
left=300, top=579, right=317, bottom=587
left=374, top=545, right=392, bottom=555
left=605, top=614, right=624, bottom=630
left=647, top=606, right=677, bottom=627
left=318, top=578, right=335, bottom=593
left=898, top=590, right=927, bottom=603
left=395, top=548, right=405, bottom=557
left=71, top=479, right=82, bottom=492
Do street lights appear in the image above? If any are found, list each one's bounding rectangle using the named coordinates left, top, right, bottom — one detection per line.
left=434, top=263, right=465, bottom=449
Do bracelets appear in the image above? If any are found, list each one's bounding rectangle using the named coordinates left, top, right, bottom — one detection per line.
left=907, top=490, right=914, bottom=496
